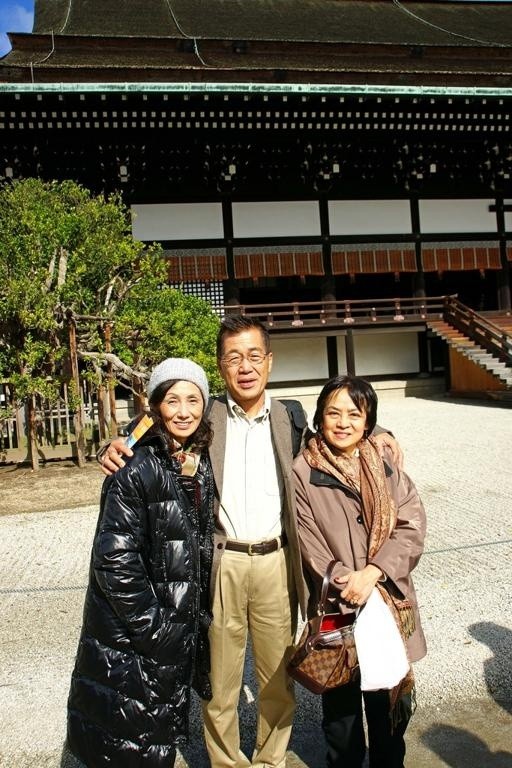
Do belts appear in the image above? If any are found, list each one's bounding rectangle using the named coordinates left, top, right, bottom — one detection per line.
left=225, top=535, right=286, bottom=556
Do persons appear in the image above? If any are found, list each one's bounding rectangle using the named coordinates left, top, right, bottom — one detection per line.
left=67, top=358, right=214, bottom=768
left=291, top=376, right=427, bottom=768
left=97, top=314, right=404, bottom=768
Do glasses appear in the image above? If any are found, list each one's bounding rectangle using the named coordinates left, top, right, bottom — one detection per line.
left=226, top=351, right=265, bottom=364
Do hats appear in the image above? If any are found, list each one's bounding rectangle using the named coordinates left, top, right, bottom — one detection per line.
left=147, top=358, right=209, bottom=413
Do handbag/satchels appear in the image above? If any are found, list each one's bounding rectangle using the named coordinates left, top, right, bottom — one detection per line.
left=287, top=561, right=358, bottom=697
